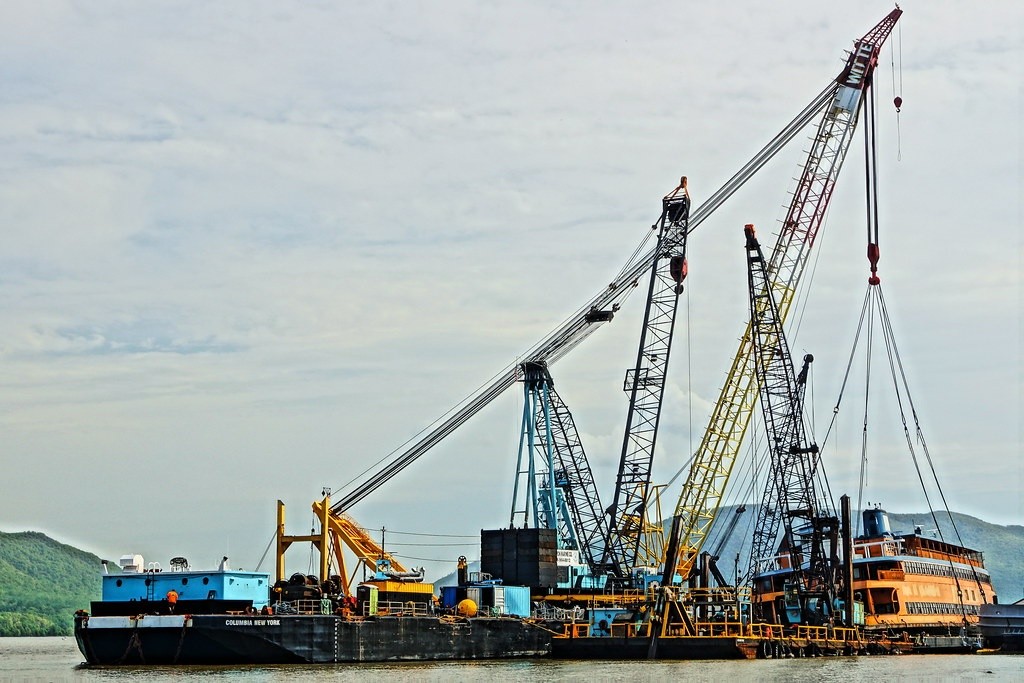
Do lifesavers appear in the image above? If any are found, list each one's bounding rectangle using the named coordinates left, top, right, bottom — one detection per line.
left=757, top=640, right=806, bottom=660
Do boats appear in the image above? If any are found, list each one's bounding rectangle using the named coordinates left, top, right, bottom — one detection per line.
left=976, top=598, right=1024, bottom=655
left=735, top=494, right=998, bottom=659
left=189, top=486, right=589, bottom=663
left=72, top=554, right=281, bottom=665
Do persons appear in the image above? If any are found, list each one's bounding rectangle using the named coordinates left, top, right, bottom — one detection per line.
left=167, top=589, right=179, bottom=611
